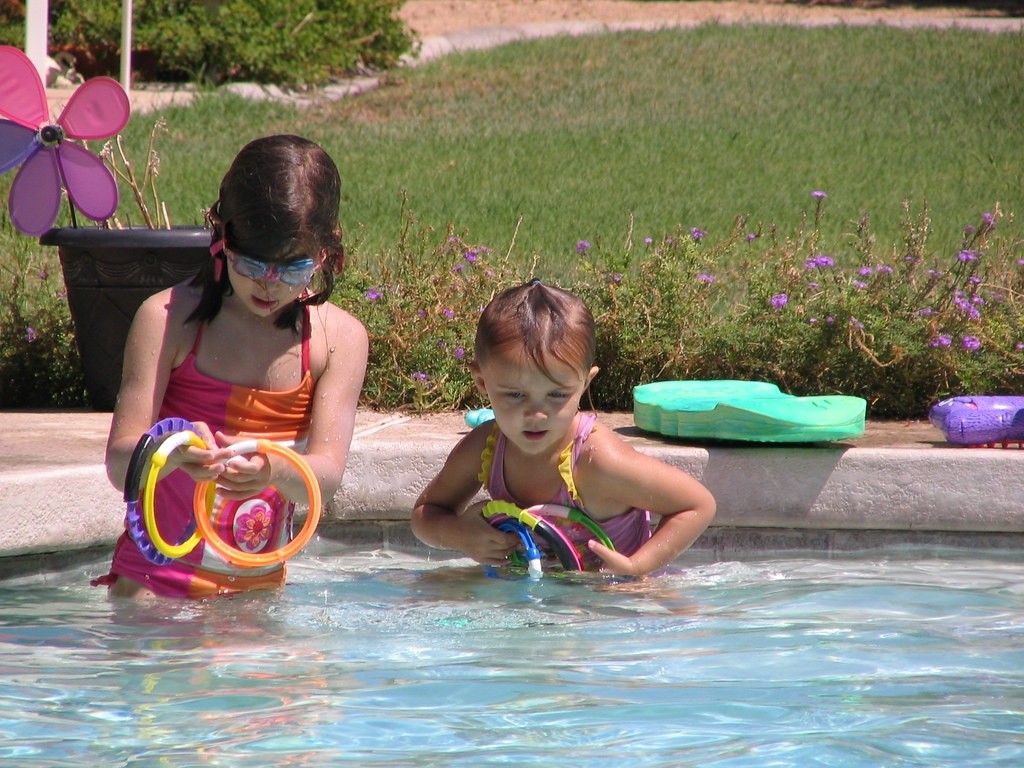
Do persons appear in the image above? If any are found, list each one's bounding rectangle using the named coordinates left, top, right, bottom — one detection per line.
left=104, top=135, right=368, bottom=614
left=410, top=279, right=717, bottom=584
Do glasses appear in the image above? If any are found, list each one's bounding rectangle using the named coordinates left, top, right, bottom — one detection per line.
left=221, top=223, right=327, bottom=286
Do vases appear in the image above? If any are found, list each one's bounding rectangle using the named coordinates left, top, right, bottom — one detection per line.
left=36, top=228, right=221, bottom=413
